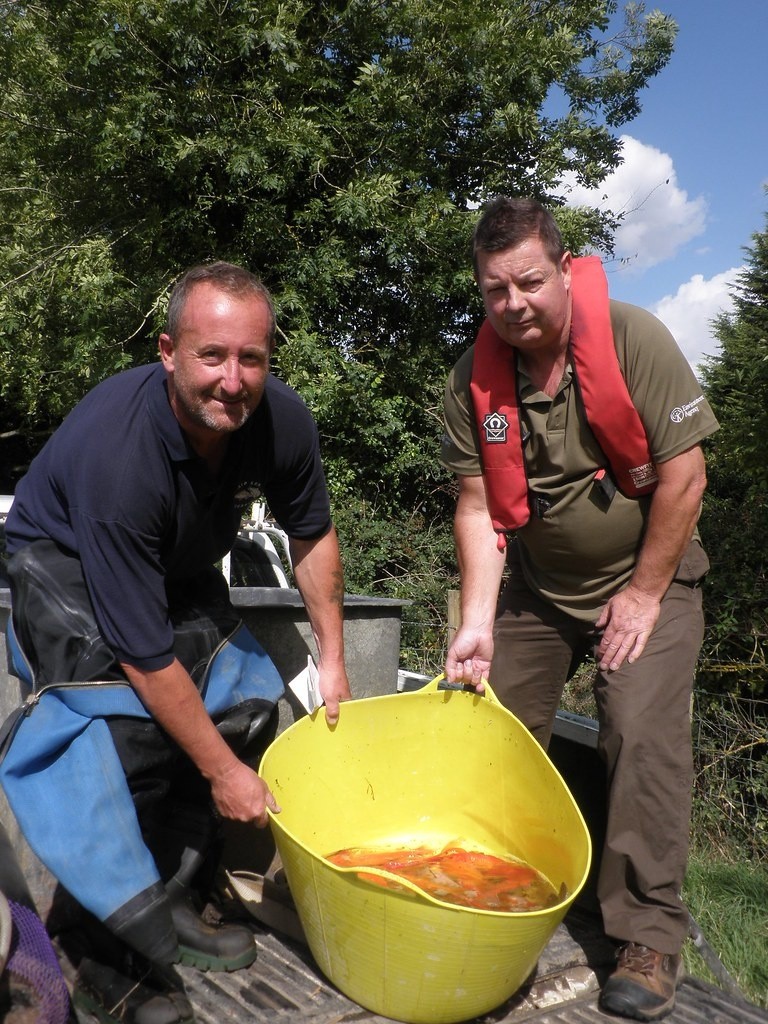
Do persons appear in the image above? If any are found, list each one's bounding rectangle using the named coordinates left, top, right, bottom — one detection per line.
left=438, top=192, right=718, bottom=1023
left=0, top=257, right=353, bottom=975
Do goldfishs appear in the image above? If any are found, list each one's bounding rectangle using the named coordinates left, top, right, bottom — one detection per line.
left=326, top=845, right=569, bottom=913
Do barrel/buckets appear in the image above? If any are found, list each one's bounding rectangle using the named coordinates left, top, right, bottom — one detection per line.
left=255, top=667, right=591, bottom=1024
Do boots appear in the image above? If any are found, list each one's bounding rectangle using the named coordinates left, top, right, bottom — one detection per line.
left=132, top=786, right=256, bottom=973
left=50, top=902, right=192, bottom=1024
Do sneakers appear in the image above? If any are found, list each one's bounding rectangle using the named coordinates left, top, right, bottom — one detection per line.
left=598, top=935, right=681, bottom=1022
